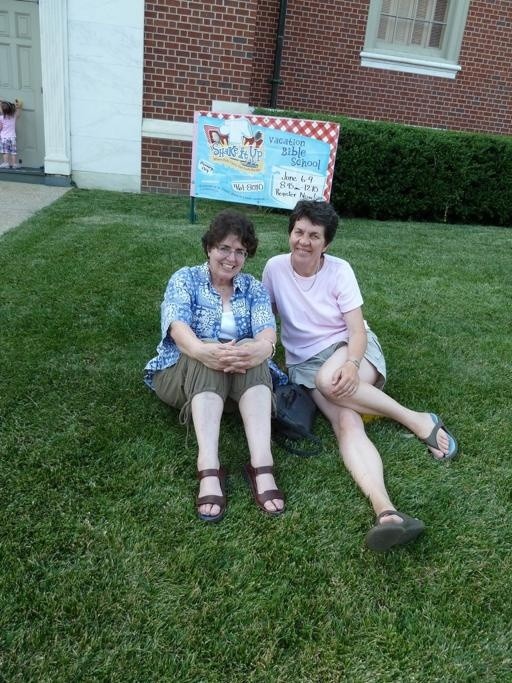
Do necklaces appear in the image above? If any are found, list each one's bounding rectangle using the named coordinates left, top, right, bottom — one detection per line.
left=292, top=255, right=320, bottom=293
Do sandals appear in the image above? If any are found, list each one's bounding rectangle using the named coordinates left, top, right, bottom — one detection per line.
left=196, top=465, right=226, bottom=522
left=242, top=458, right=285, bottom=514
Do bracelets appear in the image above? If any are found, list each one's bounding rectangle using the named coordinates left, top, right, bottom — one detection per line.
left=263, top=337, right=276, bottom=359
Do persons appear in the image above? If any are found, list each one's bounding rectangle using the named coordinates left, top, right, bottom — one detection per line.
left=0, top=98, right=21, bottom=169
left=262, top=200, right=459, bottom=553
left=142, top=207, right=290, bottom=521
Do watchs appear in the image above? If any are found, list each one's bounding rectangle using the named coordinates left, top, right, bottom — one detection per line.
left=345, top=359, right=361, bottom=370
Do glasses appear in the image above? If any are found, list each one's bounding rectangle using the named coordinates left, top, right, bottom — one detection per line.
left=215, top=241, right=248, bottom=260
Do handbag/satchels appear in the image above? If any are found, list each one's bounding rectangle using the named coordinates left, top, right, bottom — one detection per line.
left=273, top=382, right=316, bottom=440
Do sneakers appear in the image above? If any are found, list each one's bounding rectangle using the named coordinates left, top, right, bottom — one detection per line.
left=0, top=163, right=21, bottom=168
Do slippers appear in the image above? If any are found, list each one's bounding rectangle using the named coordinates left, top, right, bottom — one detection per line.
left=421, top=413, right=459, bottom=462
left=365, top=510, right=425, bottom=550
